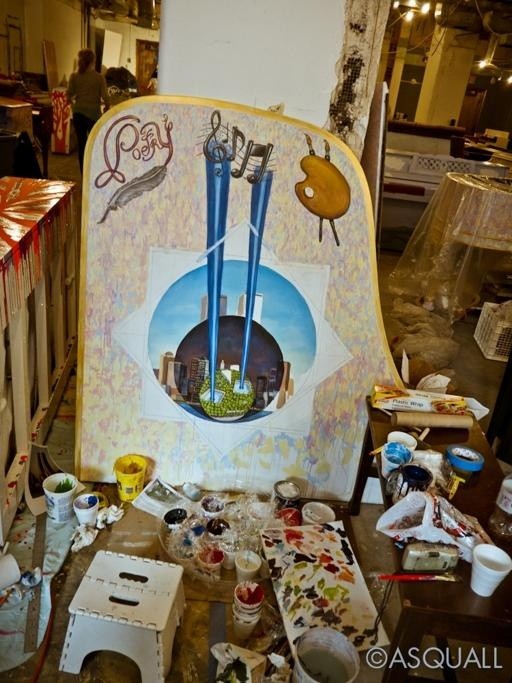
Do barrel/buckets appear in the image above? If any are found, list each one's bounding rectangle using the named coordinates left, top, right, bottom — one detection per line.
left=292, top=628, right=360, bottom=683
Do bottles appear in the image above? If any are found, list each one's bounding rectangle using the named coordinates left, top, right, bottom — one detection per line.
left=489, top=473, right=512, bottom=536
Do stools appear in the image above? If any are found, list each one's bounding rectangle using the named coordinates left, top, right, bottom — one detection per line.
left=57, top=549, right=186, bottom=683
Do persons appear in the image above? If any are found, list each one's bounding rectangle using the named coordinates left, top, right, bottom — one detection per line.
left=146, top=70, right=158, bottom=91
left=66, top=47, right=111, bottom=176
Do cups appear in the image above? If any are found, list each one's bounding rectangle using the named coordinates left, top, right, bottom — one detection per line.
left=163, top=480, right=360, bottom=681
left=469, top=543, right=511, bottom=597
left=380, top=430, right=432, bottom=504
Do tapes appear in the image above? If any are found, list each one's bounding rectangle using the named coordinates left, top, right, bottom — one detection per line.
left=447, top=445, right=485, bottom=472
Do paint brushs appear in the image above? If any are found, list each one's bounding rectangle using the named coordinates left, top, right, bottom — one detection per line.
left=377, top=574, right=461, bottom=581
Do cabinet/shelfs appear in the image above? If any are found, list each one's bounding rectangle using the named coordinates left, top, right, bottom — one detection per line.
left=381, top=148, right=509, bottom=274
left=49, top=86, right=77, bottom=155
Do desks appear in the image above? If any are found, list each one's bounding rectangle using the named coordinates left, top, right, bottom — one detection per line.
left=0, top=96, right=36, bottom=141
left=416, top=172, right=512, bottom=317
left=0, top=175, right=78, bottom=547
left=347, top=393, right=511, bottom=682
left=32, top=103, right=53, bottom=178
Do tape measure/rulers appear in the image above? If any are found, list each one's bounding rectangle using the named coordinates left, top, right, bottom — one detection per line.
left=25, top=514, right=47, bottom=653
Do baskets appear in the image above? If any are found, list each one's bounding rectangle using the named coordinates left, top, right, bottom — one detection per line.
left=473, top=301, right=511, bottom=363
